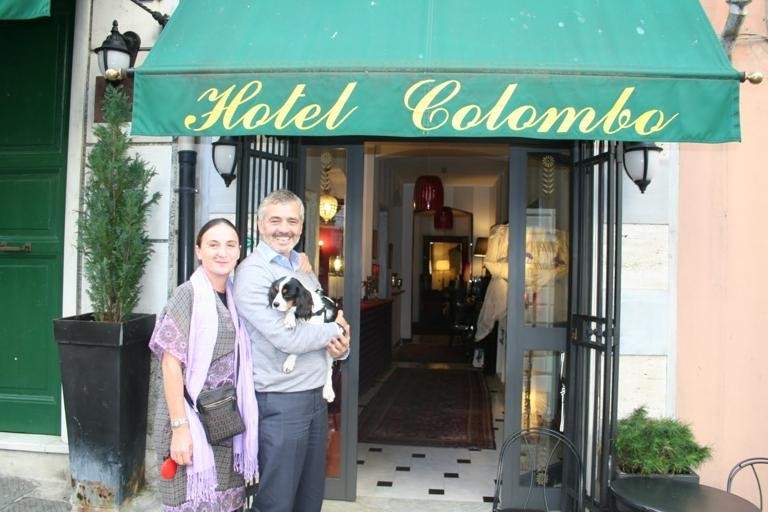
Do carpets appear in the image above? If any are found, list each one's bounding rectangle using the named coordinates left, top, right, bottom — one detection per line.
left=359, top=344, right=497, bottom=450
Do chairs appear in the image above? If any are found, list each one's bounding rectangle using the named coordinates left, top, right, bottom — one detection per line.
left=491, top=424, right=586, bottom=512
left=447, top=280, right=474, bottom=347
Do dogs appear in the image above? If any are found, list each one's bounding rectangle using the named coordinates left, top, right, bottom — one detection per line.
left=265, top=275, right=339, bottom=404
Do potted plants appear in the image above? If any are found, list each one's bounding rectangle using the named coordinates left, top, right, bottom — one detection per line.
left=49, top=81, right=161, bottom=507
left=612, top=403, right=709, bottom=483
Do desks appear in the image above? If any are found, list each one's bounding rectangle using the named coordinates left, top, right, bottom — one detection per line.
left=609, top=477, right=762, bottom=510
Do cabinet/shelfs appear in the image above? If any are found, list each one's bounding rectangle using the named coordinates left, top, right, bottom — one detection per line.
left=359, top=297, right=395, bottom=396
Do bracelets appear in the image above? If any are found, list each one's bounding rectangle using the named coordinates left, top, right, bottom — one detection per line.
left=336, top=322, right=346, bottom=336
left=170, top=417, right=188, bottom=428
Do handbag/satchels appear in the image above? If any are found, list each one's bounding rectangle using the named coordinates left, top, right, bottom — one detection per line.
left=197, top=384, right=245, bottom=444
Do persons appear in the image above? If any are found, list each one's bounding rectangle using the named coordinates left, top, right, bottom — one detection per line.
left=232, top=186, right=352, bottom=512
left=148, top=216, right=259, bottom=512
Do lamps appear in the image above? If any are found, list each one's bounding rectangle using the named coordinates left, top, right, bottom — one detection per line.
left=622, top=142, right=665, bottom=195
left=411, top=167, right=454, bottom=230
left=472, top=237, right=489, bottom=275
left=319, top=151, right=340, bottom=223
left=433, top=260, right=450, bottom=289
left=90, top=16, right=142, bottom=90
left=210, top=135, right=239, bottom=187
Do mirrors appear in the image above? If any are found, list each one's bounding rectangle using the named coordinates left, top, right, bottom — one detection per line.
left=419, top=235, right=470, bottom=329
